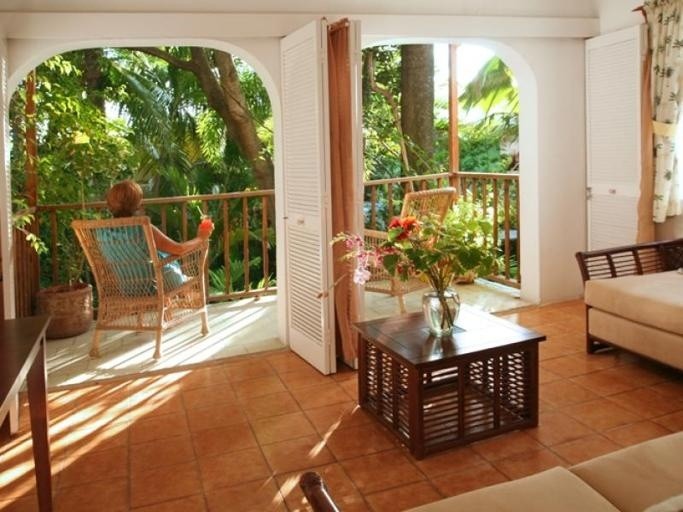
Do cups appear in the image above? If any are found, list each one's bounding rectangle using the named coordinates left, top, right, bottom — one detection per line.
left=200, top=214, right=212, bottom=226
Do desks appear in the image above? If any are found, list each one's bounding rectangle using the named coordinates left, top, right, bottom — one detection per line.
left=0, top=318, right=53, bottom=512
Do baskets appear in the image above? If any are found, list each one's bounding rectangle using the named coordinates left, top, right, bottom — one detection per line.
left=35, top=282, right=93, bottom=341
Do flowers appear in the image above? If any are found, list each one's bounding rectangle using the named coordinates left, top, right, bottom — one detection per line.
left=316, top=198, right=513, bottom=330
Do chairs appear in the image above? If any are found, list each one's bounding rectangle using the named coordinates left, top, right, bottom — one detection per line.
left=362, top=186, right=456, bottom=314
left=73, top=217, right=210, bottom=359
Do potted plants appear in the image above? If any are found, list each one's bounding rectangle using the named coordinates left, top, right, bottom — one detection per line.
left=37, top=115, right=138, bottom=342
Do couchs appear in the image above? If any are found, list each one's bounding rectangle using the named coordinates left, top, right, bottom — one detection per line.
left=299, top=425, right=683, bottom=511
left=576, top=240, right=683, bottom=376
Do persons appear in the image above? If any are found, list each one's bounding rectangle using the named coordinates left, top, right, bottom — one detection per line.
left=96, top=179, right=215, bottom=297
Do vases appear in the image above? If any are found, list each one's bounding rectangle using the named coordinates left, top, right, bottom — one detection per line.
left=420, top=290, right=460, bottom=338
